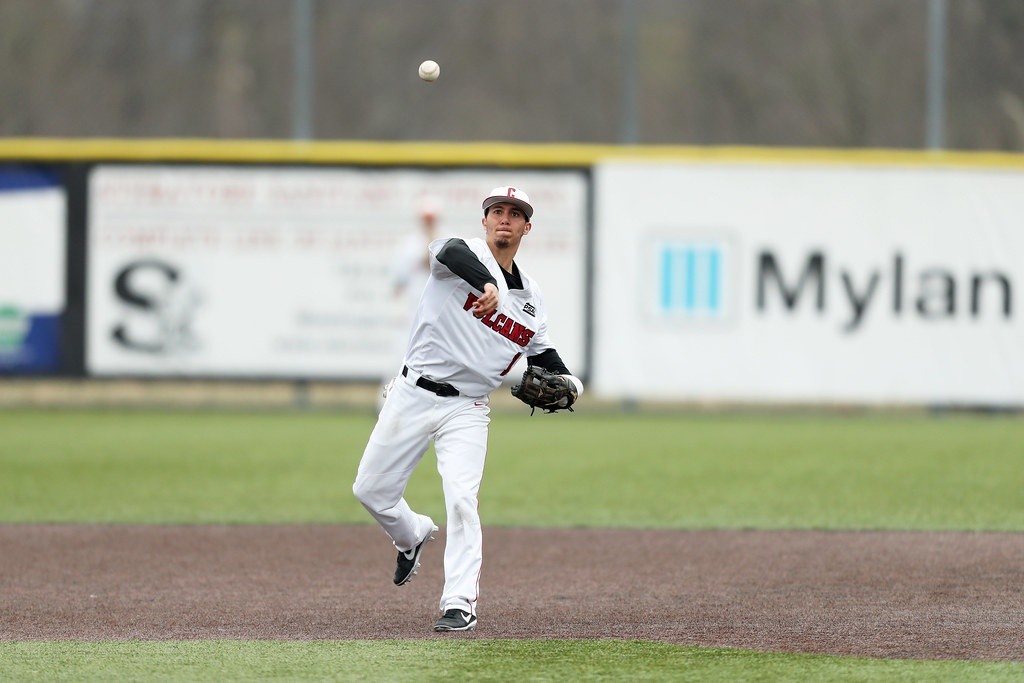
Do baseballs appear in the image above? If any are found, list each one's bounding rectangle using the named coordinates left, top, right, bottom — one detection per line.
left=418, top=58, right=441, bottom=82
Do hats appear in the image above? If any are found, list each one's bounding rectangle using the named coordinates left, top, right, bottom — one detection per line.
left=482, top=186, right=533, bottom=220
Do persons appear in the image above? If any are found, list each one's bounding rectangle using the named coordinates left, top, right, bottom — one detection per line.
left=352, top=186, right=583, bottom=631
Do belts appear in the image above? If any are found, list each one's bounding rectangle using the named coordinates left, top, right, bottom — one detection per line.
left=402, top=365, right=459, bottom=397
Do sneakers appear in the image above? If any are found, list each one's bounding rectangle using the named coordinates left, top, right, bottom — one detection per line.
left=434, top=609, right=477, bottom=631
left=393, top=522, right=439, bottom=586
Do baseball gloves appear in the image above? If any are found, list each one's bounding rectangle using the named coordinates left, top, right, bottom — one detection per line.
left=510, top=366, right=578, bottom=410
